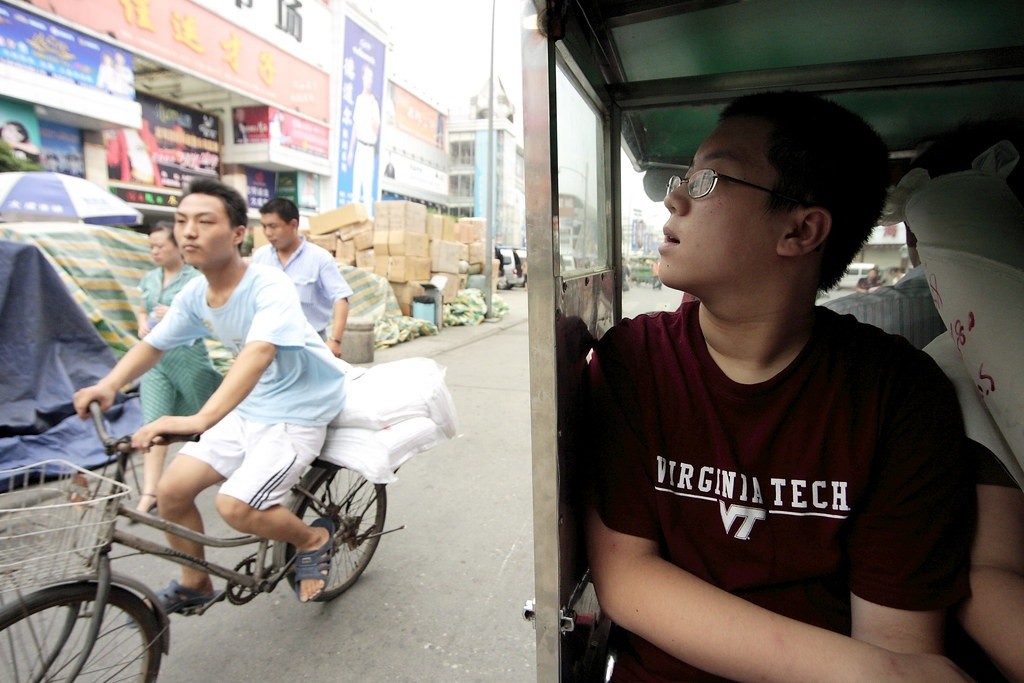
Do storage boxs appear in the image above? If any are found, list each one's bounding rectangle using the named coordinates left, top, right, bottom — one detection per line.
left=297, top=199, right=485, bottom=318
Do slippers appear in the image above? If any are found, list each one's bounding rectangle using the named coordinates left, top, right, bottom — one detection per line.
left=126, top=579, right=225, bottom=629
left=294, top=518, right=335, bottom=602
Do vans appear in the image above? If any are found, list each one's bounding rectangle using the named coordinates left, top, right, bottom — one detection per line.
left=833, top=264, right=874, bottom=291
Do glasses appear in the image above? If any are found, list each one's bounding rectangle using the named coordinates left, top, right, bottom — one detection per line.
left=666, top=168, right=813, bottom=208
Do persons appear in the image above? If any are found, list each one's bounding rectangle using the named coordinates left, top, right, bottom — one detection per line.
left=128, top=180, right=364, bottom=615
left=578, top=93, right=1022, bottom=683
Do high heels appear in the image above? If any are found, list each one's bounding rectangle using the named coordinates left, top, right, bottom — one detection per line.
left=130, top=492, right=158, bottom=523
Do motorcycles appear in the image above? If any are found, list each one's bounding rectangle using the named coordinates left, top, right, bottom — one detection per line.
left=637, top=256, right=659, bottom=289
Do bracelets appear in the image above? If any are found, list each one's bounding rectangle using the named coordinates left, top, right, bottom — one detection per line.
left=330, top=336, right=342, bottom=344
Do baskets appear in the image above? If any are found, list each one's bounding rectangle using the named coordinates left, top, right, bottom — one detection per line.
left=0, top=459, right=132, bottom=593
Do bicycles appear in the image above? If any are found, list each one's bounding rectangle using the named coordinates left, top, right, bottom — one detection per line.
left=0, top=400, right=405, bottom=683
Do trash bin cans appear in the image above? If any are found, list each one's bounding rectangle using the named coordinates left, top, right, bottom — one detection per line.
left=412, top=296, right=435, bottom=323
